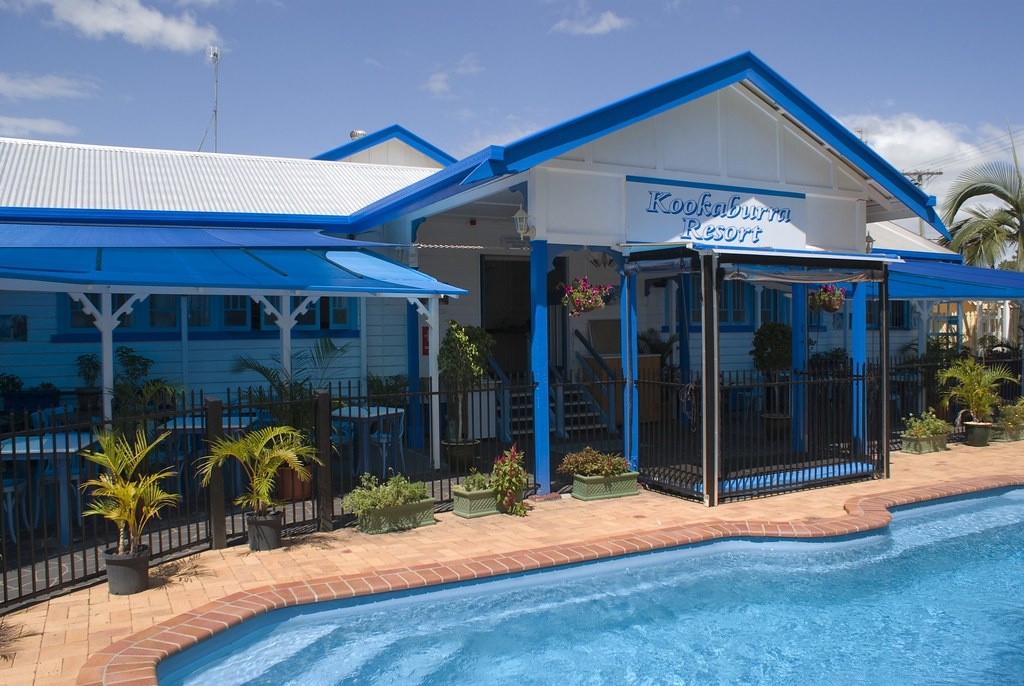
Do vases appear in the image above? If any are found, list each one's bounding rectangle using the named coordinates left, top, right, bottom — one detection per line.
left=571, top=300, right=595, bottom=312
left=823, top=299, right=841, bottom=313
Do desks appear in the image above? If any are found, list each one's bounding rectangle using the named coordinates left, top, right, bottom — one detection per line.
left=0, top=434, right=101, bottom=547
left=331, top=406, right=404, bottom=479
left=157, top=416, right=259, bottom=501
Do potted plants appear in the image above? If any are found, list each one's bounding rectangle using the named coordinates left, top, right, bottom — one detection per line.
left=934, top=357, right=1024, bottom=446
left=451, top=443, right=534, bottom=518
left=556, top=446, right=640, bottom=501
left=900, top=407, right=955, bottom=455
left=749, top=319, right=817, bottom=443
left=977, top=333, right=1024, bottom=422
left=339, top=467, right=437, bottom=535
left=901, top=333, right=973, bottom=423
left=438, top=320, right=497, bottom=474
left=367, top=371, right=408, bottom=433
left=998, top=396, right=1024, bottom=441
left=76, top=425, right=182, bottom=595
left=191, top=426, right=326, bottom=551
left=63, top=346, right=191, bottom=521
left=223, top=328, right=355, bottom=500
left=637, top=328, right=680, bottom=415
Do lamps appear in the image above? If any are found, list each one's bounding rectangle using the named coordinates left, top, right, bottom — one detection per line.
left=866, top=231, right=875, bottom=254
left=511, top=203, right=536, bottom=241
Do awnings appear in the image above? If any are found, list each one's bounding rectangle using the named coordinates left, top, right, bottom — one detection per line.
left=0, top=223, right=469, bottom=295
left=761, top=258, right=1024, bottom=299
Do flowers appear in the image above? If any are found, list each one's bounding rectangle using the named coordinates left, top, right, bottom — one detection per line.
left=559, top=276, right=616, bottom=318
left=807, top=283, right=847, bottom=312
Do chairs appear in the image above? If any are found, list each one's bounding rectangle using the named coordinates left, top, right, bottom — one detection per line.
left=30, top=407, right=102, bottom=529
left=143, top=405, right=184, bottom=464
left=868, top=370, right=900, bottom=431
left=2, top=479, right=30, bottom=543
left=370, top=413, right=405, bottom=473
left=332, top=420, right=358, bottom=476
